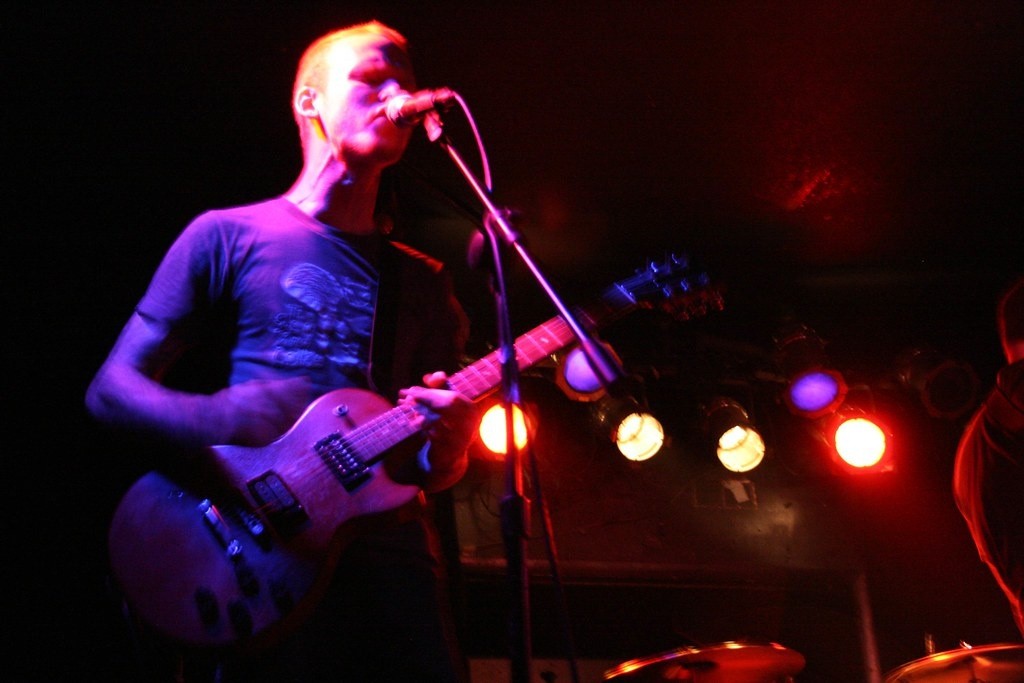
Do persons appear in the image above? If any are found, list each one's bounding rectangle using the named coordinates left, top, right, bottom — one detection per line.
left=87, top=22, right=488, bottom=683
left=950, top=281, right=1024, bottom=641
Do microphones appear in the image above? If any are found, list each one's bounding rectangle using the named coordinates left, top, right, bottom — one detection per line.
left=386, top=88, right=455, bottom=130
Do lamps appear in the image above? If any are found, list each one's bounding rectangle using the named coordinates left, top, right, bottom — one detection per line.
left=454, top=325, right=886, bottom=470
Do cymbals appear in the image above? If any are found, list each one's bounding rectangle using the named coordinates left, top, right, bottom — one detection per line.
left=882, top=642, right=1024, bottom=683
left=601, top=636, right=806, bottom=683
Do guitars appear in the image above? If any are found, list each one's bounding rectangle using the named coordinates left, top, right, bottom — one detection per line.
left=107, top=251, right=706, bottom=642
